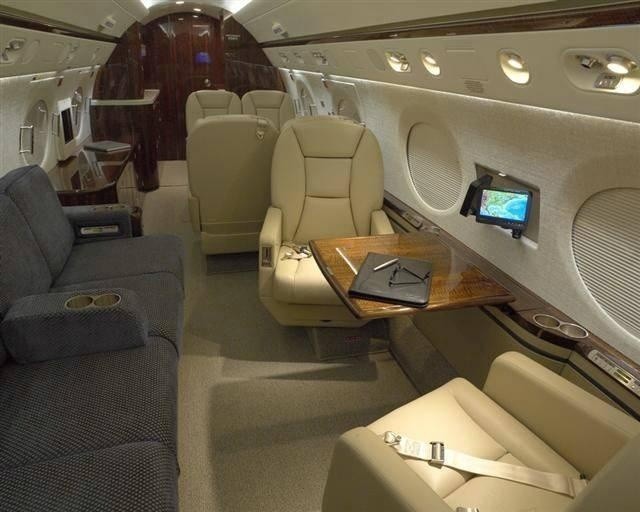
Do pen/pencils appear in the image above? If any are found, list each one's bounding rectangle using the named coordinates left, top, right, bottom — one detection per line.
left=373, top=258, right=399, bottom=271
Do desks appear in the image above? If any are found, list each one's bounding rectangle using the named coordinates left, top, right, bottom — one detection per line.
left=308, top=226, right=517, bottom=349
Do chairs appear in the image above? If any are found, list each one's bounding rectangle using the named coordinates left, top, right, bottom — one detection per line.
left=259, top=114, right=397, bottom=328
left=185, top=90, right=298, bottom=260
left=0, top=161, right=189, bottom=512
left=314, top=348, right=639, bottom=512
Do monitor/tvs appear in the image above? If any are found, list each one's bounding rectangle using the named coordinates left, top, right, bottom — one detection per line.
left=476, top=185, right=533, bottom=231
left=57, top=97, right=78, bottom=161
left=460, top=174, right=493, bottom=217
